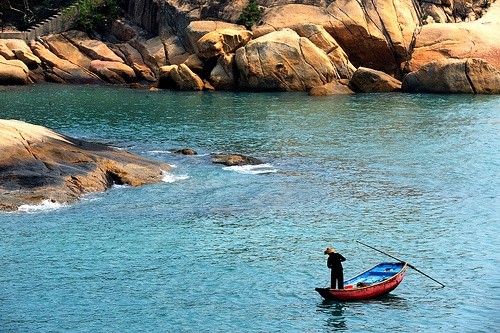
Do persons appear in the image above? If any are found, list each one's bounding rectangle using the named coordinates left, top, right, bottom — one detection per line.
left=324, top=246, right=347, bottom=289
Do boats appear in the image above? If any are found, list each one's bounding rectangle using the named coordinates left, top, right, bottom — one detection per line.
left=315, top=261, right=408, bottom=301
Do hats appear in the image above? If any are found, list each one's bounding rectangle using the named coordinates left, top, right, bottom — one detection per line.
left=324, top=248, right=334, bottom=254
